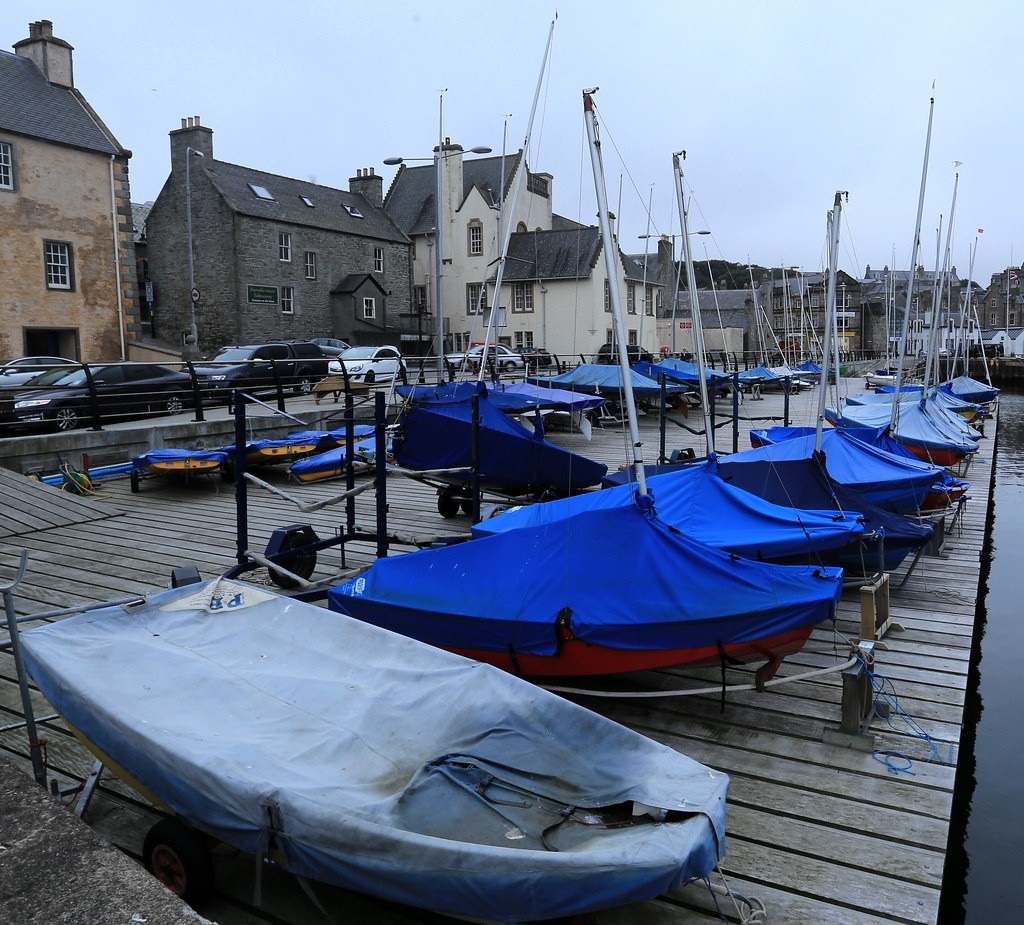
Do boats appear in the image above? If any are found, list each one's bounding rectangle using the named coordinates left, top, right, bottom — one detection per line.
left=14, top=573, right=732, bottom=922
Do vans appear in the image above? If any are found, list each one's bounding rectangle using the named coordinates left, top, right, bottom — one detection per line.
left=965, top=342, right=1004, bottom=358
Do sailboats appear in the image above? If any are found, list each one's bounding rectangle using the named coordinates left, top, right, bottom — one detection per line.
left=125, top=8, right=999, bottom=686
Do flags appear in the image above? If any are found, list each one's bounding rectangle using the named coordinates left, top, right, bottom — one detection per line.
left=978, top=228, right=983, bottom=233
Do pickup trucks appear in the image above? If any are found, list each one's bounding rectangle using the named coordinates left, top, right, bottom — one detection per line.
left=180, top=339, right=329, bottom=406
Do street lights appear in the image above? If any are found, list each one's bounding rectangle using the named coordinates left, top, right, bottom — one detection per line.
left=747, top=266, right=800, bottom=347
left=636, top=230, right=713, bottom=352
left=383, top=94, right=492, bottom=389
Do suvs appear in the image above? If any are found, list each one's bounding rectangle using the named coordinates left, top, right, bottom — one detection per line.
left=596, top=343, right=655, bottom=367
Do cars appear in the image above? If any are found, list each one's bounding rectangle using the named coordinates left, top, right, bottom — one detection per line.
left=327, top=344, right=407, bottom=385
left=515, top=347, right=552, bottom=368
left=308, top=338, right=352, bottom=361
left=0, top=360, right=200, bottom=435
left=920, top=348, right=952, bottom=358
left=0, top=356, right=86, bottom=390
left=443, top=345, right=530, bottom=372
